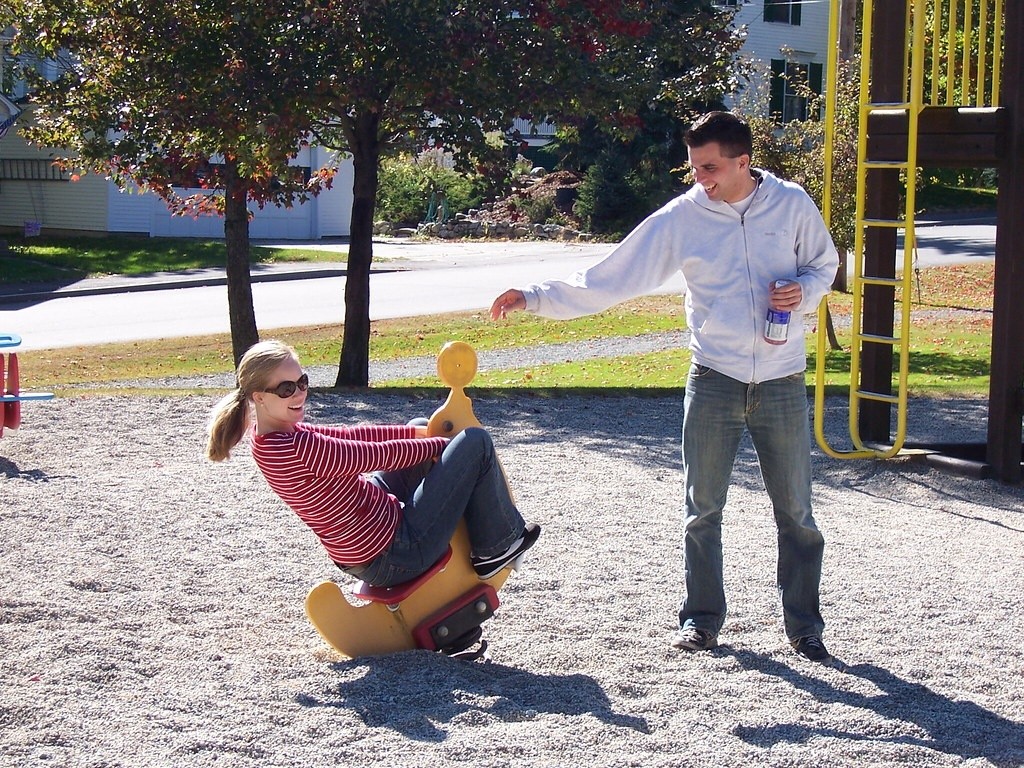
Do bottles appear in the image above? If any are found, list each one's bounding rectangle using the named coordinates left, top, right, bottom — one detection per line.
left=762, top=280, right=792, bottom=345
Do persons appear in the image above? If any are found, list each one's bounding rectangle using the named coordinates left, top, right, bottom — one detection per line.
left=489, top=110, right=839, bottom=663
left=207, top=341, right=541, bottom=588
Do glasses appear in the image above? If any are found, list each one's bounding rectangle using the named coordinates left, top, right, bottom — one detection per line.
left=258, top=373, right=309, bottom=399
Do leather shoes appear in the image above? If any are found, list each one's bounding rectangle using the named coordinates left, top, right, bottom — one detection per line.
left=334, top=561, right=373, bottom=576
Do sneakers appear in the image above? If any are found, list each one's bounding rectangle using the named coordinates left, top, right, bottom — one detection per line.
left=469, top=522, right=542, bottom=581
left=671, top=629, right=720, bottom=651
left=789, top=635, right=832, bottom=667
